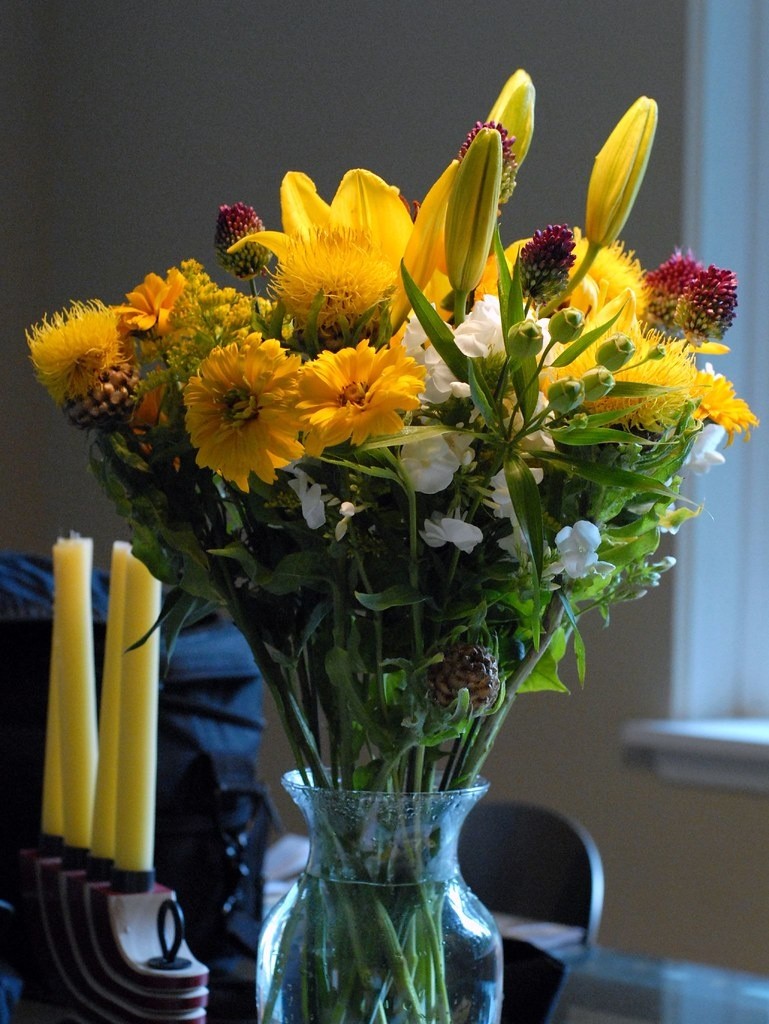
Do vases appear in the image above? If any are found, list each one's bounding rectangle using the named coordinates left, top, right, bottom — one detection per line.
left=252, top=769, right=504, bottom=1023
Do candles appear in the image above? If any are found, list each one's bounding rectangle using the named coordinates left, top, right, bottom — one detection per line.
left=31, top=532, right=164, bottom=888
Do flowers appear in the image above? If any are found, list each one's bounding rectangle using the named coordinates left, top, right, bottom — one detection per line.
left=25, top=65, right=759, bottom=717
left=405, top=65, right=415, bottom=69
